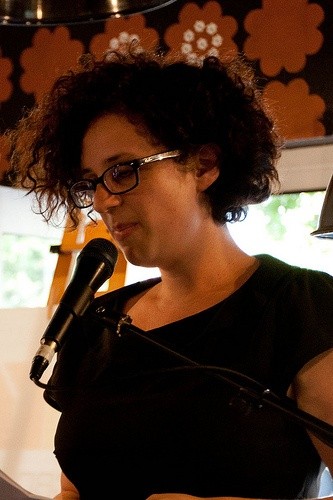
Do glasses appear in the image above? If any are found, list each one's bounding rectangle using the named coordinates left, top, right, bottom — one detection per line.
left=64, top=149, right=179, bottom=210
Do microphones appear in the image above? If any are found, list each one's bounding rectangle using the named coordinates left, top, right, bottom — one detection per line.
left=30, top=238, right=119, bottom=382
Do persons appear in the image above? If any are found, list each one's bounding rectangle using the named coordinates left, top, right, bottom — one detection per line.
left=6, top=42, right=333, bottom=499
